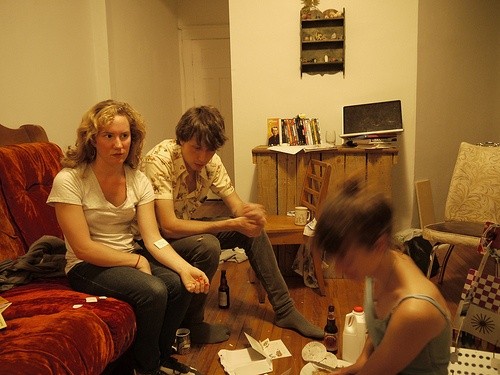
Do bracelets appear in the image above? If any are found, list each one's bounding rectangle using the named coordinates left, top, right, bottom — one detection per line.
left=134, top=254, right=140, bottom=268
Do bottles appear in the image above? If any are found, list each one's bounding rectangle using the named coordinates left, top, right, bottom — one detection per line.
left=341, top=306, right=368, bottom=363
left=323, top=305, right=339, bottom=354
left=217, top=270, right=231, bottom=309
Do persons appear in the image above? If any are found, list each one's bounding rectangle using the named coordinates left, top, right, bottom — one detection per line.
left=136, top=105, right=326, bottom=345
left=46, top=100, right=211, bottom=375
left=267, top=127, right=280, bottom=147
left=313, top=170, right=452, bottom=375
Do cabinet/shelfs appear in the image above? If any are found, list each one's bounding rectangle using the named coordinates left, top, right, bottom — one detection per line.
left=252, top=145, right=400, bottom=277
left=300, top=8, right=345, bottom=79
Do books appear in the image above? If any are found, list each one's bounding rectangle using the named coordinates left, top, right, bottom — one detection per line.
left=267, top=117, right=321, bottom=147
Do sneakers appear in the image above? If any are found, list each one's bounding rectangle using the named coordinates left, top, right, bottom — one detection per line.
left=130, top=355, right=200, bottom=375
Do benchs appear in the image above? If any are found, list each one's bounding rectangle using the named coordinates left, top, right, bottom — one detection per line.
left=0, top=124, right=137, bottom=375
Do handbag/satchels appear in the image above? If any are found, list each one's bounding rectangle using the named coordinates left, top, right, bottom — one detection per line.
left=480, top=222, right=500, bottom=250
left=405, top=237, right=440, bottom=278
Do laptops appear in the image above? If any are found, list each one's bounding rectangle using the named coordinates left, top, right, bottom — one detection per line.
left=340, top=99, right=403, bottom=138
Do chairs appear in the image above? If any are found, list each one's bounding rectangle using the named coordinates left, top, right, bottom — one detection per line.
left=258, top=158, right=332, bottom=303
left=422, top=142, right=500, bottom=284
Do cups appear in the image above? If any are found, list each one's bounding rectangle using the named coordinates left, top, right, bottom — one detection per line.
left=326, top=130, right=336, bottom=145
left=176, top=328, right=191, bottom=355
left=294, top=206, right=310, bottom=226
